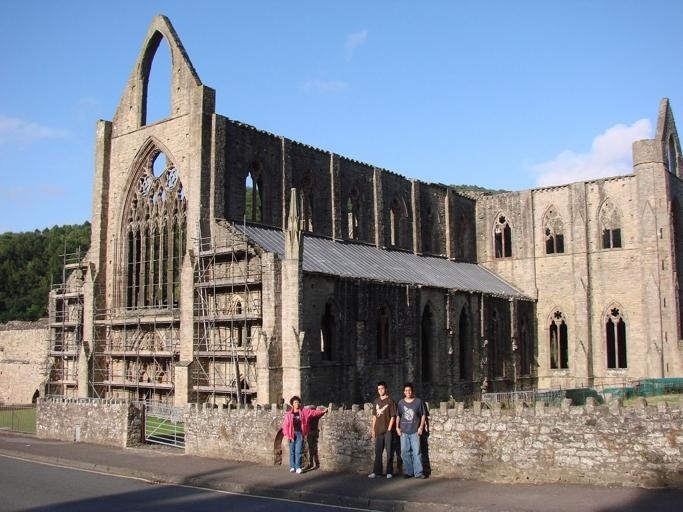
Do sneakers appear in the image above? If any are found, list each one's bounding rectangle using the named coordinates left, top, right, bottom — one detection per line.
left=290, top=468, right=303, bottom=474
left=387, top=474, right=392, bottom=479
left=368, top=473, right=382, bottom=478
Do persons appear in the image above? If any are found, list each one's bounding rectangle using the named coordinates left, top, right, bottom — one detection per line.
left=283, top=397, right=329, bottom=473
left=367, top=381, right=398, bottom=478
left=397, top=383, right=429, bottom=479
